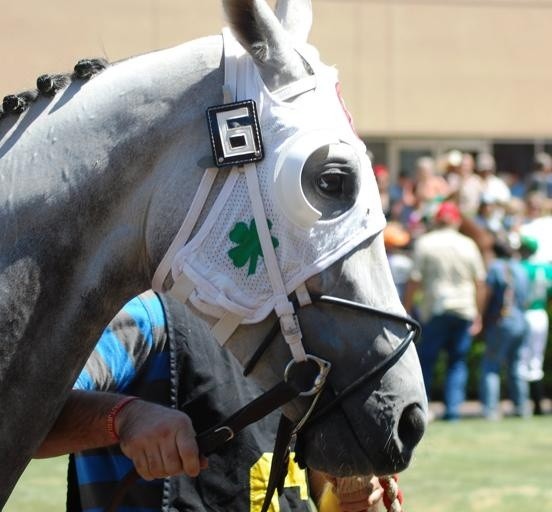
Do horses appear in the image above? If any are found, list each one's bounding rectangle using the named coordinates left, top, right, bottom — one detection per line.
left=0, top=0, right=432, bottom=512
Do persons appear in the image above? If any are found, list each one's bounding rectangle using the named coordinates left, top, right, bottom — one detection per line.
left=364, top=149, right=552, bottom=425
left=31, top=287, right=403, bottom=511
